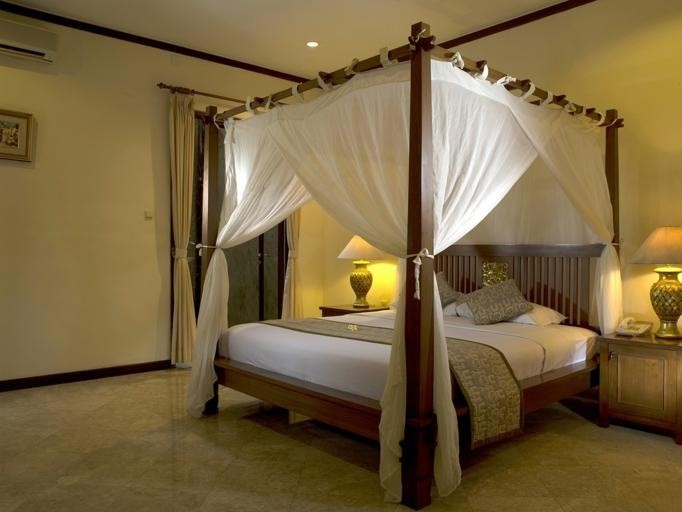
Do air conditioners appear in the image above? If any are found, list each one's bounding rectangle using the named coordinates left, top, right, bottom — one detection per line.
left=0, top=18, right=57, bottom=65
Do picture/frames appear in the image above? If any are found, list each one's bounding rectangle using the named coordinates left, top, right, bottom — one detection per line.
left=0, top=108, right=33, bottom=163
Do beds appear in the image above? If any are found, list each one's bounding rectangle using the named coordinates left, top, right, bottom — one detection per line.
left=184, top=23, right=625, bottom=509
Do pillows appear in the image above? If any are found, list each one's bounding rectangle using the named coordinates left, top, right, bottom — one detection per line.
left=463, top=279, right=534, bottom=325
left=437, top=272, right=460, bottom=309
left=443, top=301, right=459, bottom=316
left=456, top=300, right=567, bottom=326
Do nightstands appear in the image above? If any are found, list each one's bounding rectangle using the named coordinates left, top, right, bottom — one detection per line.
left=595, top=333, right=682, bottom=445
left=319, top=304, right=390, bottom=317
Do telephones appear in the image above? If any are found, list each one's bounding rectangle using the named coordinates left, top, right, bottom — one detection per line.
left=615, top=316, right=653, bottom=335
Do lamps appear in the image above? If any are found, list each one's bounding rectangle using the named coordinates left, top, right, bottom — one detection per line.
left=337, top=234, right=384, bottom=308
left=628, top=226, right=682, bottom=338
